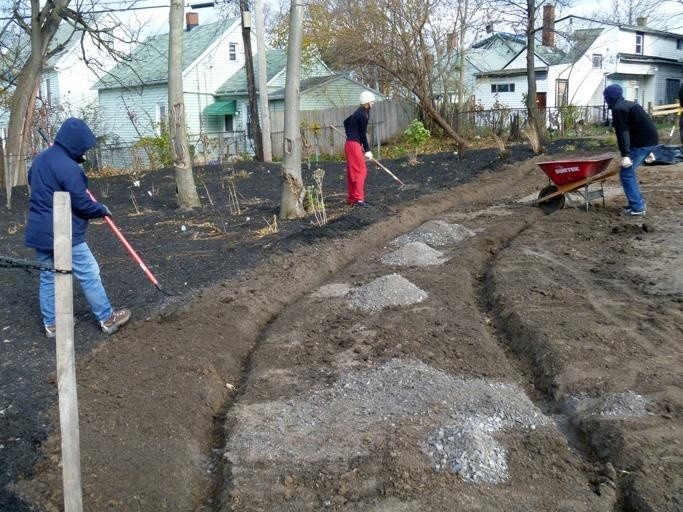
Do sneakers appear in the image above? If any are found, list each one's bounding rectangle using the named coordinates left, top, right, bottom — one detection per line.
left=44, top=319, right=76, bottom=337
left=99, top=307, right=132, bottom=334
left=623, top=200, right=647, bottom=216
left=353, top=200, right=366, bottom=206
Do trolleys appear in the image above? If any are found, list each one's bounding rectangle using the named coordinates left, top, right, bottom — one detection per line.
left=532, top=149, right=632, bottom=214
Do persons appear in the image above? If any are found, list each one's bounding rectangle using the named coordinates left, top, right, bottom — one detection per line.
left=24, top=118, right=133, bottom=339
left=343, top=90, right=376, bottom=208
left=603, top=84, right=658, bottom=216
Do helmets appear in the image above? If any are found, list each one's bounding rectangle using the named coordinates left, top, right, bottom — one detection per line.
left=359, top=91, right=375, bottom=105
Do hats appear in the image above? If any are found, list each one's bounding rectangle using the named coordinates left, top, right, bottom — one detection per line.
left=604, top=85, right=622, bottom=99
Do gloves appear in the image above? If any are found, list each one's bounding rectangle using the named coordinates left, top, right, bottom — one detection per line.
left=621, top=156, right=633, bottom=168
left=364, top=151, right=372, bottom=160
left=101, top=203, right=112, bottom=218
